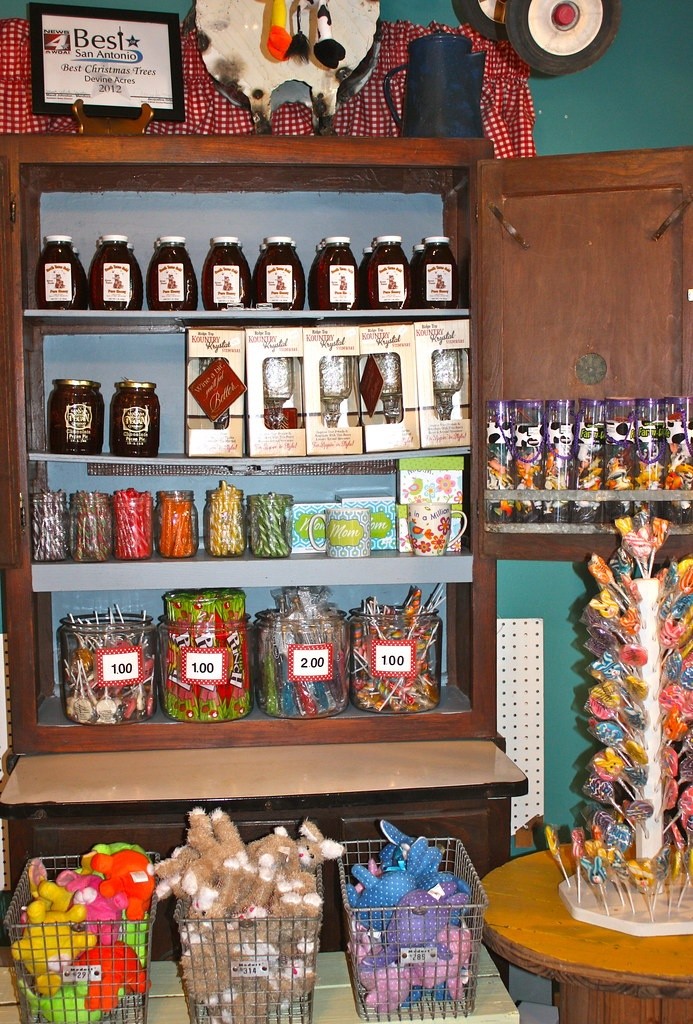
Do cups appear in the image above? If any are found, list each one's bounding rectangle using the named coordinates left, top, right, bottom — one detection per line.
left=309, top=507, right=372, bottom=558
left=407, top=502, right=467, bottom=556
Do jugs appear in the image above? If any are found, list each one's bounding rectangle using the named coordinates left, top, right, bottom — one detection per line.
left=384, top=30, right=486, bottom=138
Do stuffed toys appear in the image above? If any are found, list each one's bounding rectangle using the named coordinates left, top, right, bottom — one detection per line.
left=156, top=807, right=344, bottom=1024
left=12, top=842, right=155, bottom=1024
left=345, top=820, right=471, bottom=1013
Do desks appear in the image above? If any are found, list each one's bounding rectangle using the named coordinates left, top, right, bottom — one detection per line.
left=476, top=838, right=692, bottom=1024
left=0, top=941, right=521, bottom=1024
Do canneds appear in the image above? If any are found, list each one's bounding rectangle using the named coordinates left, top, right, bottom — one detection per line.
left=48, top=378, right=161, bottom=458
left=36, top=235, right=460, bottom=311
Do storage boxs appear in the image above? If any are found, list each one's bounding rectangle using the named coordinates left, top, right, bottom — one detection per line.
left=342, top=495, right=397, bottom=551
left=396, top=458, right=465, bottom=553
left=287, top=496, right=340, bottom=557
left=184, top=318, right=471, bottom=458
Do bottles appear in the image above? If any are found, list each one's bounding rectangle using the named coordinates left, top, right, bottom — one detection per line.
left=255, top=609, right=349, bottom=720
left=36, top=235, right=459, bottom=312
left=372, top=352, right=402, bottom=423
left=203, top=489, right=245, bottom=557
left=154, top=488, right=198, bottom=559
left=263, top=356, right=294, bottom=430
left=198, top=357, right=229, bottom=429
left=109, top=381, right=161, bottom=457
left=246, top=494, right=293, bottom=557
left=432, top=349, right=463, bottom=420
left=348, top=607, right=442, bottom=713
left=58, top=614, right=155, bottom=726
left=46, top=379, right=104, bottom=455
left=319, top=356, right=351, bottom=428
left=68, top=493, right=111, bottom=562
left=155, top=613, right=256, bottom=723
left=112, top=491, right=153, bottom=560
left=29, top=492, right=69, bottom=560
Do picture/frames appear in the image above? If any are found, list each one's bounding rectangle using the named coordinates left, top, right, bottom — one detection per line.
left=29, top=1, right=186, bottom=122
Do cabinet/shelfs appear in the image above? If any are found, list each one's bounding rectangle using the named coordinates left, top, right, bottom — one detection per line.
left=0, top=133, right=527, bottom=962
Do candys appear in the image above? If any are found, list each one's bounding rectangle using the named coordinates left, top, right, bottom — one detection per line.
left=348, top=583, right=445, bottom=713
left=259, top=586, right=350, bottom=719
left=58, top=601, right=153, bottom=727
left=543, top=514, right=693, bottom=924
left=32, top=480, right=296, bottom=558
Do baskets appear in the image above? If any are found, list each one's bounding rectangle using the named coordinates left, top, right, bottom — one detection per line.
left=337, top=834, right=488, bottom=1020
left=169, top=817, right=323, bottom=1023
left=4, top=849, right=161, bottom=1023
